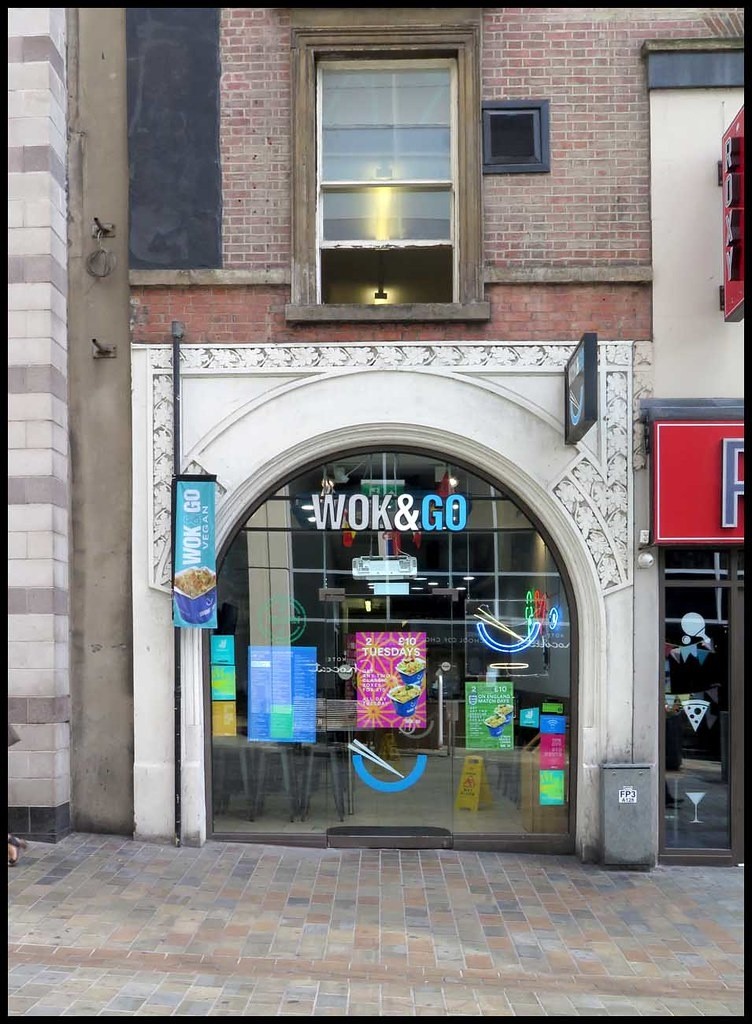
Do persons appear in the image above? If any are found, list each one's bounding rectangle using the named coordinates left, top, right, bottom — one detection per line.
left=8, top=724, right=27, bottom=866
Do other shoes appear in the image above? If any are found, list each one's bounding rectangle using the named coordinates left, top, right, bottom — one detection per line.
left=664, top=797, right=685, bottom=809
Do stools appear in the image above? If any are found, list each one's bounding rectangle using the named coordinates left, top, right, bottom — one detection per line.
left=212, top=744, right=345, bottom=821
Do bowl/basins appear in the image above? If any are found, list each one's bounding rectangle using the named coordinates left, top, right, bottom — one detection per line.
left=387, top=684, right=421, bottom=717
left=484, top=704, right=513, bottom=737
left=174, top=566, right=216, bottom=624
left=395, top=658, right=426, bottom=688
